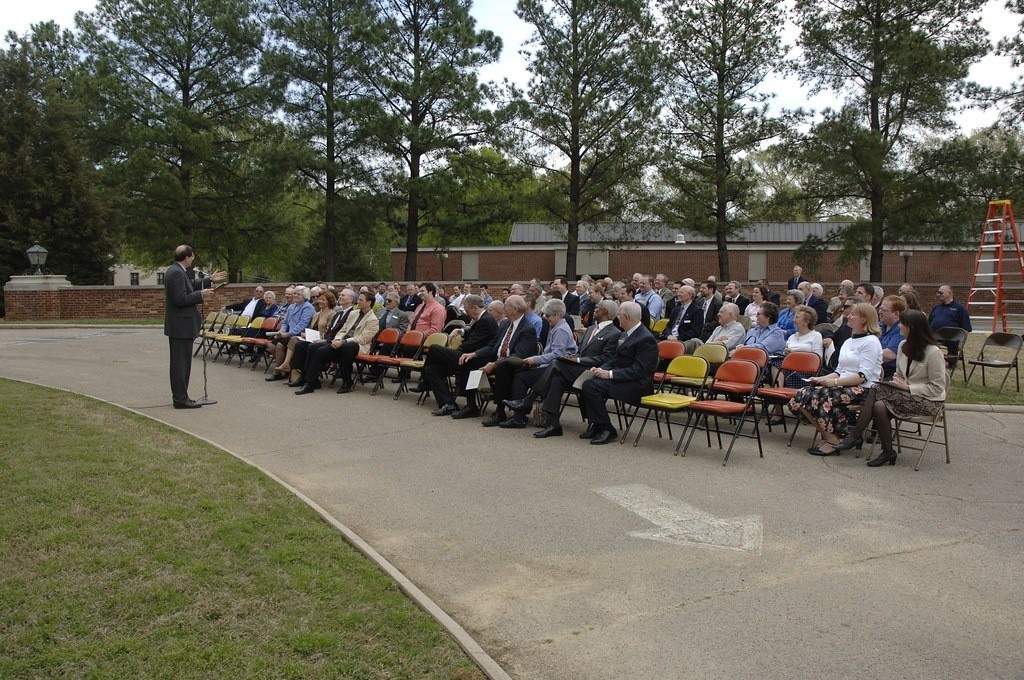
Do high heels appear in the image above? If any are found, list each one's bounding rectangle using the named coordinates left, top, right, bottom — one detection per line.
left=807, top=438, right=897, bottom=467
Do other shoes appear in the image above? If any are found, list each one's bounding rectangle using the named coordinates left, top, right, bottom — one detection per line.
left=765, top=417, right=783, bottom=426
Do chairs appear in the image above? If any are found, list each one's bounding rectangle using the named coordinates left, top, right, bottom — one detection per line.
left=192, top=311, right=286, bottom=373
left=318, top=315, right=1023, bottom=471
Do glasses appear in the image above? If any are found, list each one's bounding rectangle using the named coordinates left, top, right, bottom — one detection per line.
left=311, top=295, right=319, bottom=298
left=937, top=290, right=949, bottom=294
left=190, top=255, right=195, bottom=260
left=843, top=304, right=852, bottom=310
left=386, top=299, right=392, bottom=302
left=879, top=305, right=895, bottom=313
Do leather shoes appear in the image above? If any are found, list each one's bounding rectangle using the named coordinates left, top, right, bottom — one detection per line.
left=220, top=343, right=619, bottom=444
left=174, top=399, right=202, bottom=409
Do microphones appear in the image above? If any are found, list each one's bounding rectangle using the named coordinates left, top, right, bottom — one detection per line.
left=193, top=268, right=209, bottom=276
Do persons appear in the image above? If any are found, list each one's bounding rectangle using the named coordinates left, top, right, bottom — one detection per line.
left=220, top=265, right=972, bottom=467
left=164, top=245, right=228, bottom=409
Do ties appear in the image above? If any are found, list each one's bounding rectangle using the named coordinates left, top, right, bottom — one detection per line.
left=459, top=295, right=466, bottom=307
left=617, top=334, right=629, bottom=349
left=411, top=304, right=426, bottom=330
left=732, top=299, right=735, bottom=304
left=803, top=301, right=806, bottom=305
left=500, top=323, right=513, bottom=358
left=326, top=311, right=344, bottom=338
left=376, top=310, right=389, bottom=334
left=588, top=325, right=599, bottom=344
left=676, top=306, right=684, bottom=325
left=793, top=277, right=797, bottom=288
left=407, top=296, right=412, bottom=308
left=702, top=302, right=707, bottom=312
